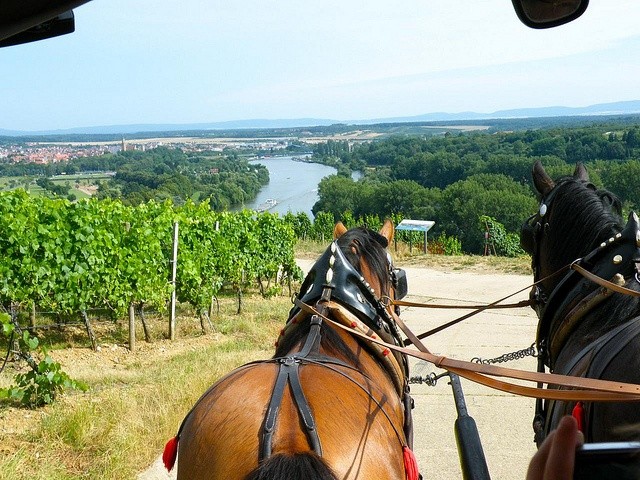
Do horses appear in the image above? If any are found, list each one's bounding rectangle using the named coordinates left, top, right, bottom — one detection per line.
left=176, top=218, right=414, bottom=480
left=519, top=160, right=640, bottom=480
